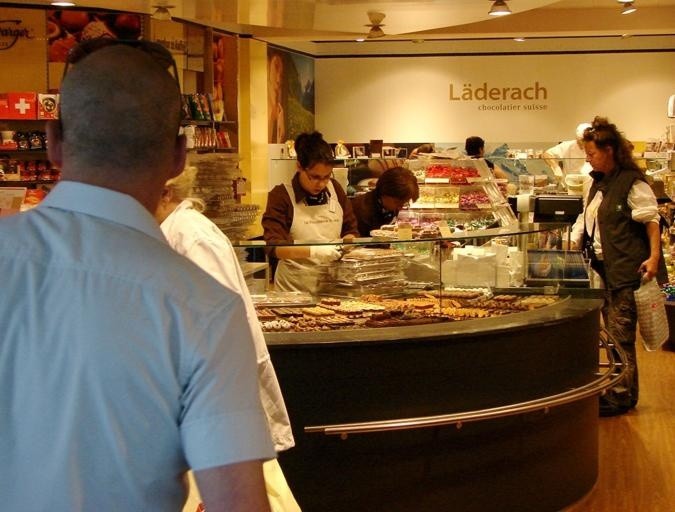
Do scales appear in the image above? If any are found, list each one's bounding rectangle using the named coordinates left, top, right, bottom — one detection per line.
left=524, top=194, right=590, bottom=287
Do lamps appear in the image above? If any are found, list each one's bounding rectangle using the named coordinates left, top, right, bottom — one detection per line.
left=149, top=4, right=172, bottom=22
left=488, top=0, right=513, bottom=16
left=621, top=0, right=641, bottom=18
left=366, top=23, right=387, bottom=38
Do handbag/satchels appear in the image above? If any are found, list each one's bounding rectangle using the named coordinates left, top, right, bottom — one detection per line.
left=633, top=276, right=670, bottom=353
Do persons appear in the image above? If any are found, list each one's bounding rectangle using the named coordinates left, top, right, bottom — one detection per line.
left=349, top=165, right=420, bottom=236
left=0, top=39, right=282, bottom=512
left=562, top=113, right=663, bottom=418
left=262, top=131, right=359, bottom=295
left=465, top=136, right=508, bottom=180
left=542, top=121, right=592, bottom=176
left=267, top=48, right=292, bottom=143
left=154, top=160, right=297, bottom=512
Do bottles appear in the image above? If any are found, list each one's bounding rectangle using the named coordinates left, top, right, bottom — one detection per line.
left=515, top=150, right=547, bottom=197
left=546, top=230, right=562, bottom=250
left=0, top=159, right=62, bottom=182
left=194, top=127, right=216, bottom=148
left=179, top=91, right=211, bottom=120
left=16, top=132, right=49, bottom=150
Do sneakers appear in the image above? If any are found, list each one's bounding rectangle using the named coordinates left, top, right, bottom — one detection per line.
left=598, top=394, right=638, bottom=417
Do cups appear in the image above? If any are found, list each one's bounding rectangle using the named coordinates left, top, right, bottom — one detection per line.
left=397, top=222, right=412, bottom=240
left=47, top=22, right=60, bottom=39
left=1, top=130, right=16, bottom=144
left=531, top=262, right=551, bottom=277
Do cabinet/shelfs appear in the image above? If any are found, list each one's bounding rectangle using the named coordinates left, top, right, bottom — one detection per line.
left=0, top=113, right=239, bottom=187
left=379, top=156, right=519, bottom=253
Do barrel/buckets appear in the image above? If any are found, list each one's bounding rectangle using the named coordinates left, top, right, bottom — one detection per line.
left=565, top=173, right=587, bottom=196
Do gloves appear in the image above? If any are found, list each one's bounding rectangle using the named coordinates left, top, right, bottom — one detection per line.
left=309, top=245, right=343, bottom=264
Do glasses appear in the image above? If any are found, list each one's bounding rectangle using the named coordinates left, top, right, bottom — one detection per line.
left=304, top=168, right=335, bottom=183
left=61, top=36, right=183, bottom=99
